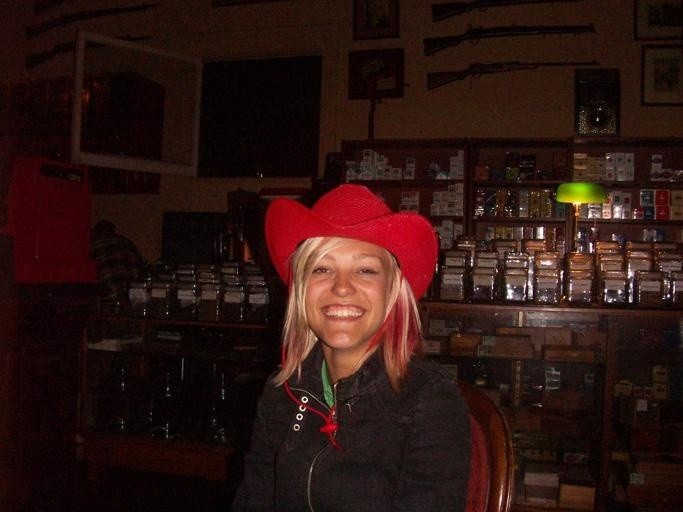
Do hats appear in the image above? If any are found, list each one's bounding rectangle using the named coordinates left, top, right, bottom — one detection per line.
left=261, top=185, right=441, bottom=305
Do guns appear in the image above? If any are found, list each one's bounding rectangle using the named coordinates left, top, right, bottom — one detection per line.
left=431, top=0, right=578, bottom=23
left=422, top=22, right=596, bottom=59
left=427, top=58, right=600, bottom=90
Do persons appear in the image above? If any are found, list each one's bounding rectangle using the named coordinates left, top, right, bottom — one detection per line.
left=232, top=183, right=471, bottom=512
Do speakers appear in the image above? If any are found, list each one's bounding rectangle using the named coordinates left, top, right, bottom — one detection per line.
left=574, top=67, right=622, bottom=136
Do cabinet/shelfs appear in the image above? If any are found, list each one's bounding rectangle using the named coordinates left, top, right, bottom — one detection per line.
left=69, top=137, right=682, bottom=507
